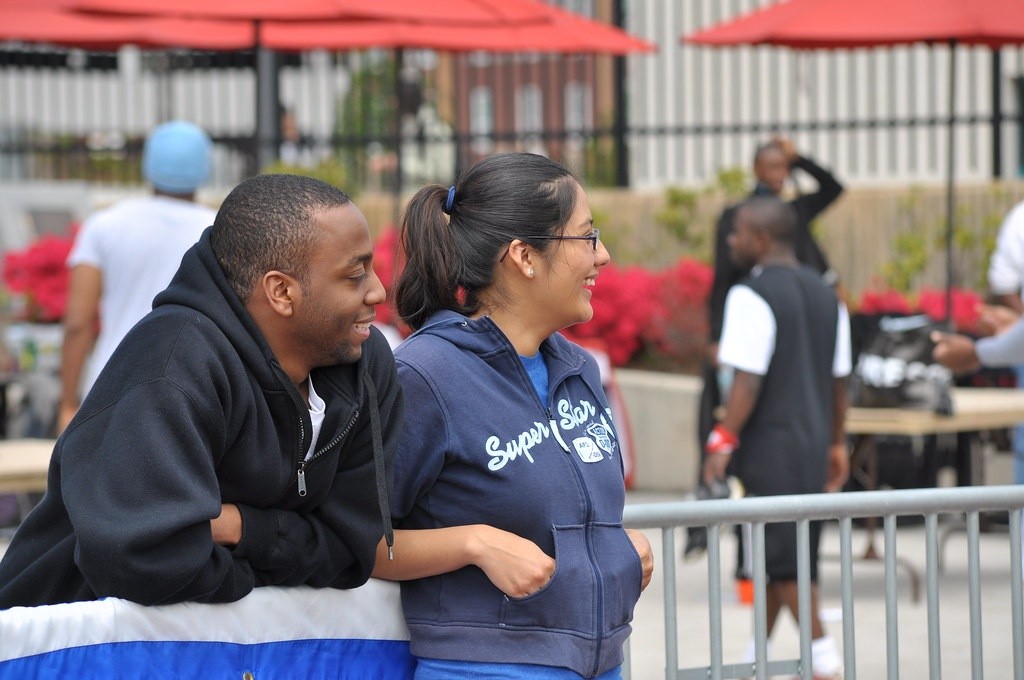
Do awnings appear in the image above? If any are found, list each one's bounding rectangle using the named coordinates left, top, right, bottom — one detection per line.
left=0, top=0, right=662, bottom=56
left=681, top=0, right=1023, bottom=49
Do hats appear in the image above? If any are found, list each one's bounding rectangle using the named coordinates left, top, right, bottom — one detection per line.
left=141, top=122, right=214, bottom=192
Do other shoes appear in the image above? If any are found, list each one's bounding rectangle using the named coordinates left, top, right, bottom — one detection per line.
left=787, top=638, right=841, bottom=680
left=746, top=639, right=770, bottom=664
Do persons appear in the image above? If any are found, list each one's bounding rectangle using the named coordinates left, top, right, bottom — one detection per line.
left=931, top=200, right=1024, bottom=486
left=0, top=174, right=405, bottom=612
left=685, top=139, right=853, bottom=680
left=58, top=120, right=216, bottom=435
left=372, top=154, right=655, bottom=680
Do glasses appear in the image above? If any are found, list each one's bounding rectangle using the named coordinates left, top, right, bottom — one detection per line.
left=499, top=228, right=600, bottom=264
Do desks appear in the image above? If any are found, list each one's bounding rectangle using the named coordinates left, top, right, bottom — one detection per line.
left=0, top=437, right=57, bottom=527
left=816, top=387, right=1024, bottom=601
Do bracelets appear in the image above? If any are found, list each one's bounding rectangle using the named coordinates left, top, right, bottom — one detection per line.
left=704, top=422, right=740, bottom=455
